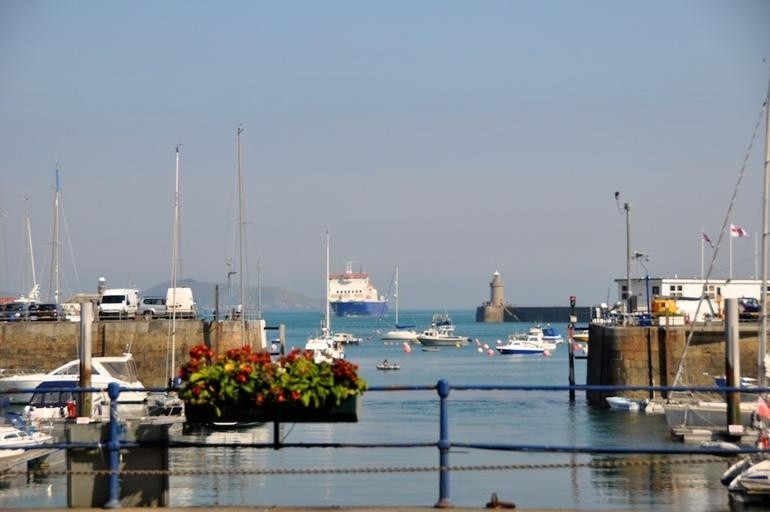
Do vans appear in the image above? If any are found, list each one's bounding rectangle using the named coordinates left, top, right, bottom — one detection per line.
left=167, top=287, right=195, bottom=318
left=100, top=288, right=138, bottom=320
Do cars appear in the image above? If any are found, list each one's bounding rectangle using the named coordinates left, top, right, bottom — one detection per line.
left=737, top=297, right=761, bottom=316
left=0, top=302, right=66, bottom=321
left=137, top=298, right=165, bottom=320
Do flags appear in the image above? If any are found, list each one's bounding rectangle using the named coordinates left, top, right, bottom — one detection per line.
left=730, top=226, right=748, bottom=238
left=702, top=232, right=711, bottom=244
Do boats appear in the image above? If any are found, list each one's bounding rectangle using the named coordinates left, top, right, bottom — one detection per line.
left=0, top=352, right=188, bottom=474
left=381, top=263, right=470, bottom=346
left=327, top=263, right=391, bottom=317
left=496, top=323, right=564, bottom=354
left=376, top=359, right=400, bottom=369
left=304, top=227, right=362, bottom=364
left=605, top=376, right=770, bottom=503
left=573, top=330, right=589, bottom=342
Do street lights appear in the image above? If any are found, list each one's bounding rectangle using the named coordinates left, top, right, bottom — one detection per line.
left=615, top=190, right=650, bottom=313
